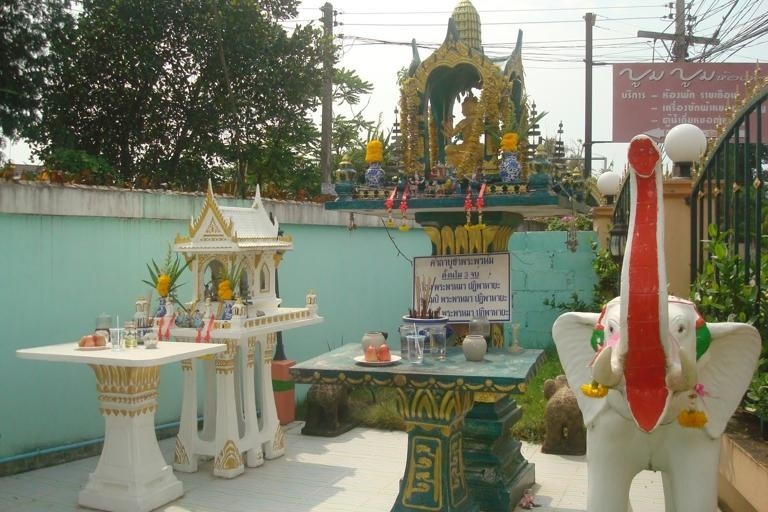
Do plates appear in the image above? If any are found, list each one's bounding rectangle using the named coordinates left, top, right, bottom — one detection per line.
left=72, top=344, right=110, bottom=349
left=354, top=354, right=401, bottom=367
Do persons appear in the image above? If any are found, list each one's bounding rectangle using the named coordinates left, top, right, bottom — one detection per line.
left=443, top=93, right=485, bottom=165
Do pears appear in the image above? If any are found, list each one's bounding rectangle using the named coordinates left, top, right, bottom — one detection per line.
left=79, top=334, right=106, bottom=347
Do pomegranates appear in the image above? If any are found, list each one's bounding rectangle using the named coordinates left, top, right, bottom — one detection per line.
left=378, top=345, right=390, bottom=361
left=365, top=344, right=377, bottom=362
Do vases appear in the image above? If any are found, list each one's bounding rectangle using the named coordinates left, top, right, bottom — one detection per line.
left=498, top=152, right=522, bottom=183
left=364, top=162, right=386, bottom=188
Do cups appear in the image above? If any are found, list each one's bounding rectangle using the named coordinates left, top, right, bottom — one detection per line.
left=124, top=320, right=138, bottom=348
left=400, top=325, right=419, bottom=360
left=407, top=334, right=426, bottom=366
left=430, top=327, right=447, bottom=362
left=109, top=327, right=126, bottom=355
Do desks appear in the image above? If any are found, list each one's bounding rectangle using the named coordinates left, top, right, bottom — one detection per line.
left=14, top=337, right=228, bottom=512
left=288, top=341, right=549, bottom=511
